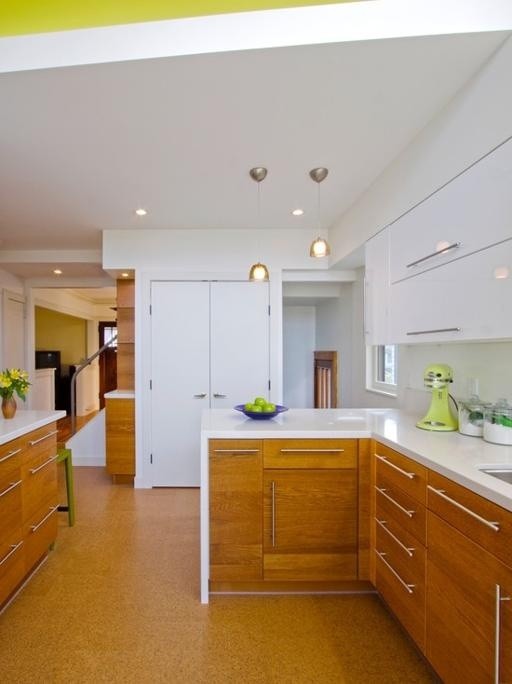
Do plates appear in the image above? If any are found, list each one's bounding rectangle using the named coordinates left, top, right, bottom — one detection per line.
left=235, top=403, right=288, bottom=418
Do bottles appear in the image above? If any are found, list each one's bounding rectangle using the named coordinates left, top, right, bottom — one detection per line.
left=457, top=393, right=492, bottom=436
left=483, top=397, right=512, bottom=445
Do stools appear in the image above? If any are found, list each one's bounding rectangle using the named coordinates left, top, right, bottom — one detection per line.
left=56, top=449, right=74, bottom=528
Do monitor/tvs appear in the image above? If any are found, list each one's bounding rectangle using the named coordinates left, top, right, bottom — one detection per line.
left=35, top=351, right=61, bottom=376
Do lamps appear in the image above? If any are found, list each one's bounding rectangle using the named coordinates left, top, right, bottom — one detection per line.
left=309, top=167, right=330, bottom=257
left=249, top=166, right=273, bottom=282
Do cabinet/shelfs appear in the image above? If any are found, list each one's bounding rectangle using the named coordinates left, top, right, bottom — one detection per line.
left=373, top=441, right=430, bottom=665
left=363, top=225, right=393, bottom=348
left=207, top=434, right=373, bottom=596
left=23, top=419, right=58, bottom=583
left=392, top=133, right=512, bottom=346
left=111, top=277, right=135, bottom=392
left=0, top=436, right=24, bottom=615
left=104, top=388, right=135, bottom=483
left=427, top=465, right=512, bottom=684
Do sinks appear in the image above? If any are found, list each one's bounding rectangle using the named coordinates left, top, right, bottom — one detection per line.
left=479, top=466, right=512, bottom=488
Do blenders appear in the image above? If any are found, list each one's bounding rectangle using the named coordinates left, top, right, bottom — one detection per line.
left=415, top=364, right=459, bottom=433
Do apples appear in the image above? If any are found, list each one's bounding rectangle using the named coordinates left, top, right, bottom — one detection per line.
left=245, top=397, right=277, bottom=413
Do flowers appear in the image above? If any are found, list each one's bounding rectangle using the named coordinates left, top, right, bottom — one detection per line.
left=0, top=367, right=30, bottom=403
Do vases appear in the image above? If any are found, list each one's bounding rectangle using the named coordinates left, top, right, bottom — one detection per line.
left=2, top=392, right=19, bottom=420
